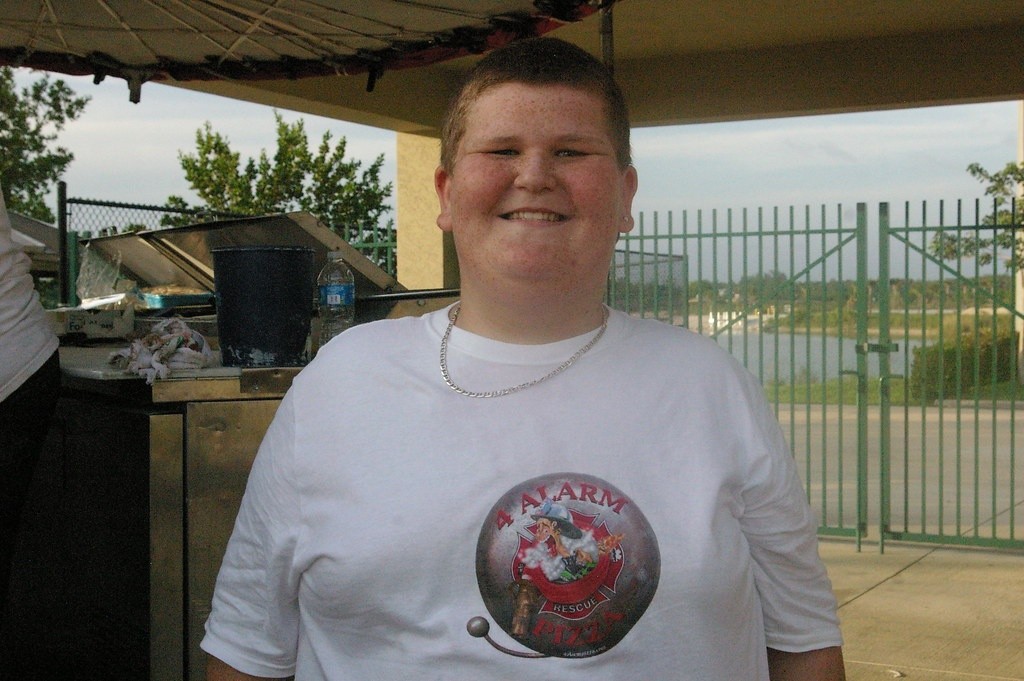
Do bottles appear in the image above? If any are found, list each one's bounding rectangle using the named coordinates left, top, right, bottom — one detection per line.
left=317, top=252, right=355, bottom=349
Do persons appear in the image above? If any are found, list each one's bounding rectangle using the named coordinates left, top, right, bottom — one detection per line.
left=197, top=35, right=846, bottom=680
left=438, top=299, right=609, bottom=399
left=0, top=187, right=61, bottom=614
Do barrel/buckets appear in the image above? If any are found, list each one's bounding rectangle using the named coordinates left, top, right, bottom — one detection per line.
left=210, top=245, right=313, bottom=369
